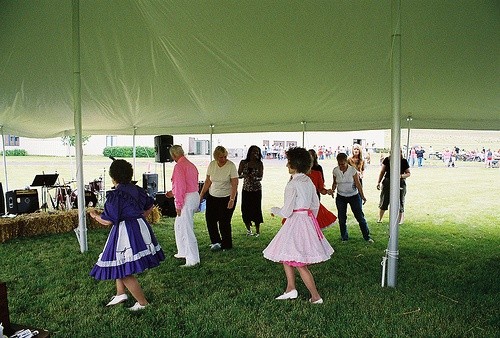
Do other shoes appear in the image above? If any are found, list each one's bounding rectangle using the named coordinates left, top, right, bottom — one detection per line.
left=128, top=302, right=146, bottom=311
left=309, top=298, right=323, bottom=303
left=369, top=239, right=374, bottom=243
left=275, top=289, right=298, bottom=299
left=106, top=294, right=127, bottom=307
left=174, top=254, right=185, bottom=258
left=209, top=243, right=221, bottom=247
left=247, top=231, right=251, bottom=234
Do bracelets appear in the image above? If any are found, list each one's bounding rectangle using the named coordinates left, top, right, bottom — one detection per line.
left=230, top=199, right=234, bottom=201
left=401, top=174, right=402, bottom=178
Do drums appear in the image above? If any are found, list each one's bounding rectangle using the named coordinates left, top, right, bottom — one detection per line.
left=93, top=180, right=102, bottom=192
left=70, top=188, right=97, bottom=208
left=88, top=182, right=98, bottom=193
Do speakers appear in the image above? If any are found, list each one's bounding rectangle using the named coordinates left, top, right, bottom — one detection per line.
left=6, top=188, right=39, bottom=216
left=154, top=134, right=173, bottom=163
left=143, top=174, right=159, bottom=193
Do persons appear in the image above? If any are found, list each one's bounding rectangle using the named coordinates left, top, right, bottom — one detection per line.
left=376, top=148, right=410, bottom=224
left=262, top=147, right=335, bottom=304
left=238, top=145, right=264, bottom=237
left=308, top=149, right=325, bottom=202
left=282, top=167, right=337, bottom=228
left=348, top=144, right=364, bottom=209
left=331, top=153, right=374, bottom=243
left=261, top=144, right=351, bottom=160
left=91, top=159, right=166, bottom=310
left=409, top=145, right=500, bottom=168
left=200, top=146, right=239, bottom=251
left=166, top=145, right=200, bottom=267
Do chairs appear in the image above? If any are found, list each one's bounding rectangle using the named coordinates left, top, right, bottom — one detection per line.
left=0, top=279, right=50, bottom=338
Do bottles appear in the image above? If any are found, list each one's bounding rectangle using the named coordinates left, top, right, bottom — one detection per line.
left=198, top=181, right=204, bottom=193
